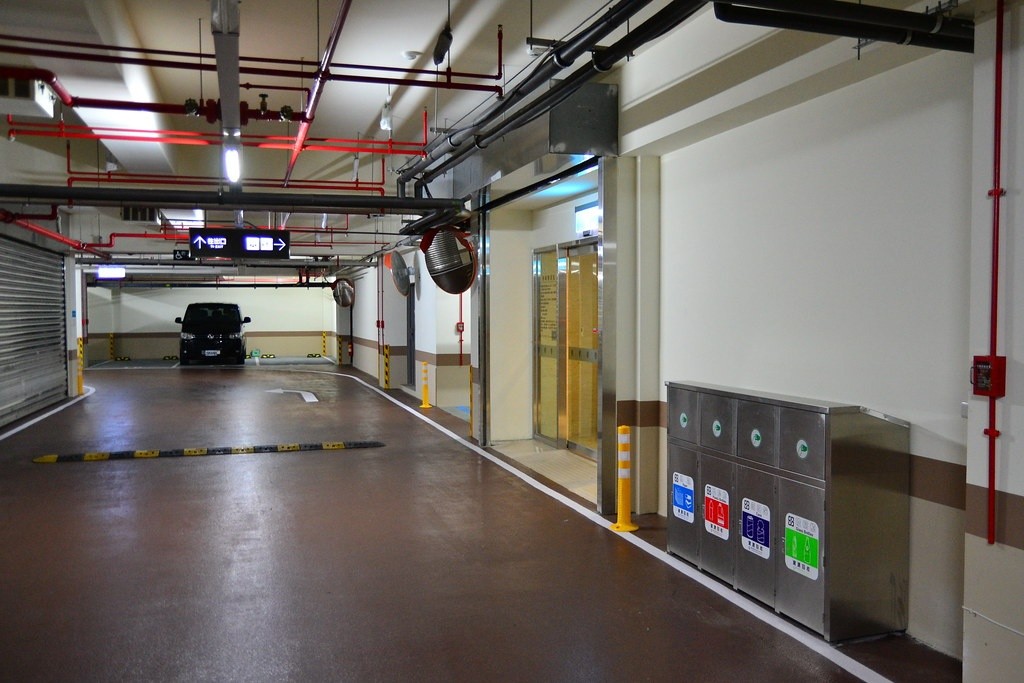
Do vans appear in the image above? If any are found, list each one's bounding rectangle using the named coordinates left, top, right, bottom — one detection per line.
left=175, top=302, right=251, bottom=365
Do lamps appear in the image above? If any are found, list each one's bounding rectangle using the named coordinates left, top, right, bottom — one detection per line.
left=378, top=103, right=394, bottom=132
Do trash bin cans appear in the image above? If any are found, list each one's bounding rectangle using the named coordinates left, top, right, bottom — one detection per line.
left=664, top=378, right=912, bottom=642
